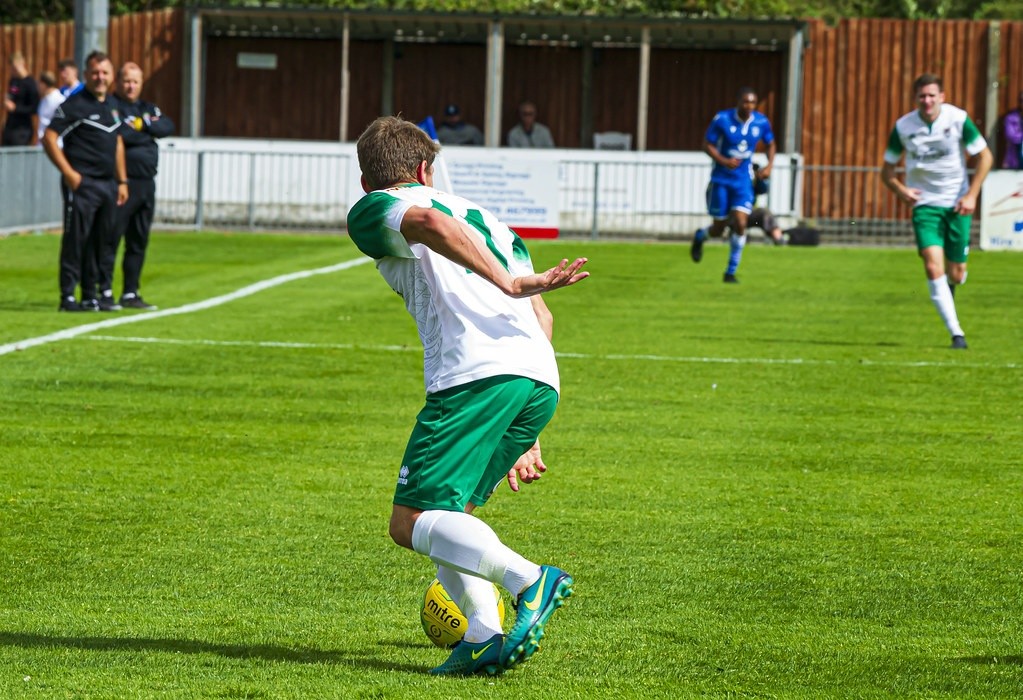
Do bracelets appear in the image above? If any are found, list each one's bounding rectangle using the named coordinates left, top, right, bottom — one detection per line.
left=117, top=179, right=129, bottom=185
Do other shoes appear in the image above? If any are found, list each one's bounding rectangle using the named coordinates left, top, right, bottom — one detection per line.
left=774, top=234, right=790, bottom=245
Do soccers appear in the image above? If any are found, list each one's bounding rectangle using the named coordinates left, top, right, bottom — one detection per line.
left=419, top=577, right=505, bottom=649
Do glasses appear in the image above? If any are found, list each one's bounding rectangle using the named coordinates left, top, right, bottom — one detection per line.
left=522, top=110, right=535, bottom=116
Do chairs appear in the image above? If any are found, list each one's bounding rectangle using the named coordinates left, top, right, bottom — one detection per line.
left=592, top=131, right=633, bottom=150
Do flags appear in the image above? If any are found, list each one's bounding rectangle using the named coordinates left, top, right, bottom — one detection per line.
left=418, top=116, right=438, bottom=140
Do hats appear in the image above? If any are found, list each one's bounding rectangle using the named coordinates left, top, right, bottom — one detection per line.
left=444, top=104, right=460, bottom=116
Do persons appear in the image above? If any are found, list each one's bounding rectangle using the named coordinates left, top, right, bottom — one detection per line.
left=508, top=101, right=555, bottom=148
left=0, top=51, right=41, bottom=146
left=692, top=88, right=775, bottom=284
left=435, top=104, right=485, bottom=146
left=881, top=74, right=995, bottom=349
left=42, top=50, right=127, bottom=313
left=344, top=116, right=590, bottom=676
left=101, top=61, right=176, bottom=311
left=996, top=89, right=1023, bottom=170
left=728, top=162, right=789, bottom=246
left=37, top=71, right=67, bottom=146
left=57, top=60, right=86, bottom=97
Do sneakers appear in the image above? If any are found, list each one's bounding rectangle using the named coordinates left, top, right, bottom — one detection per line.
left=432, top=634, right=504, bottom=679
left=949, top=284, right=955, bottom=294
left=60, top=295, right=100, bottom=311
left=952, top=335, right=967, bottom=348
left=119, top=297, right=158, bottom=310
left=724, top=273, right=738, bottom=283
left=499, top=565, right=574, bottom=672
left=692, top=229, right=704, bottom=263
left=99, top=294, right=123, bottom=311
